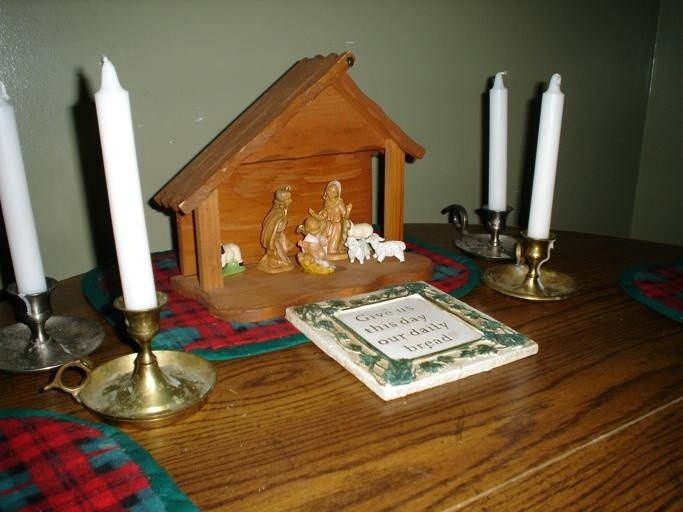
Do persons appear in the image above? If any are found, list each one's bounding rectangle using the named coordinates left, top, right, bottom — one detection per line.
left=308, top=180, right=352, bottom=257
left=297, top=216, right=336, bottom=275
left=256, top=189, right=293, bottom=273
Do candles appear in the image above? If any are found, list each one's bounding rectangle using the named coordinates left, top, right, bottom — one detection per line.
left=487, top=70, right=509, bottom=212
left=524, top=72, right=566, bottom=239
left=0, top=80, right=47, bottom=295
left=92, top=55, right=158, bottom=311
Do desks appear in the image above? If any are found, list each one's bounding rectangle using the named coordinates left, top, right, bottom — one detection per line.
left=0, top=223, right=679, bottom=511
